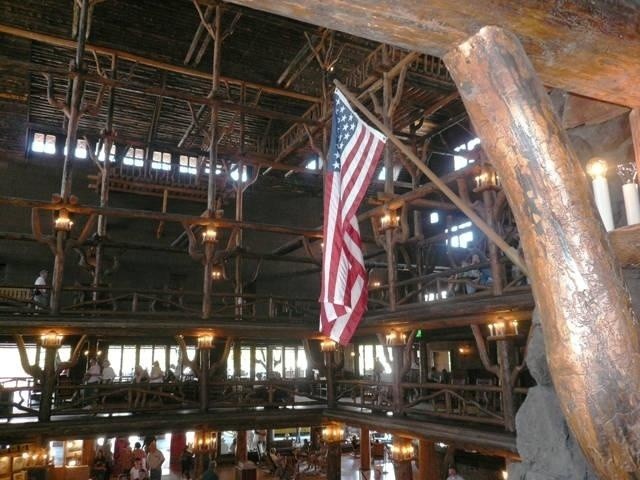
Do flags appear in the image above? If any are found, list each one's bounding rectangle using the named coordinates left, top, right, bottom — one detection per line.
left=317, top=87, right=390, bottom=346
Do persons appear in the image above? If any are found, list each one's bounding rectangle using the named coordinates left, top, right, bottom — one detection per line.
left=428, top=366, right=450, bottom=402
left=91, top=435, right=380, bottom=480
left=463, top=253, right=481, bottom=293
left=376, top=357, right=385, bottom=382
left=446, top=463, right=464, bottom=480
left=27, top=270, right=49, bottom=315
left=61, top=353, right=178, bottom=409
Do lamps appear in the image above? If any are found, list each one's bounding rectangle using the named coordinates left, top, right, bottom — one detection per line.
left=198, top=437, right=218, bottom=452
left=22, top=450, right=47, bottom=465
left=55, top=218, right=73, bottom=230
left=474, top=173, right=499, bottom=187
left=202, top=230, right=217, bottom=241
left=617, top=163, right=639, bottom=226
left=322, top=429, right=344, bottom=440
left=384, top=333, right=407, bottom=346
left=320, top=342, right=336, bottom=352
left=585, top=158, right=614, bottom=231
left=487, top=319, right=519, bottom=337
left=389, top=446, right=416, bottom=460
left=381, top=215, right=400, bottom=226
left=40, top=334, right=63, bottom=345
left=197, top=336, right=213, bottom=347
